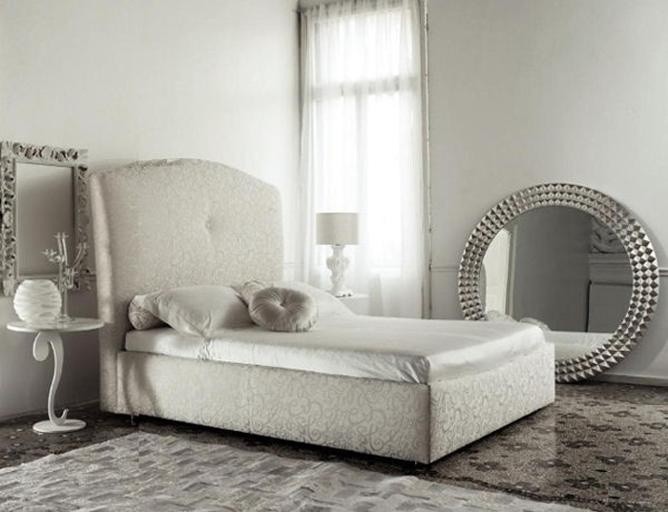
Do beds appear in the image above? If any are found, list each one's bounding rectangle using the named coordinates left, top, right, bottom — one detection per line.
left=88, top=155, right=556, bottom=466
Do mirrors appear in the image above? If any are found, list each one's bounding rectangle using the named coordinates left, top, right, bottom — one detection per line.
left=0, top=140, right=91, bottom=298
left=457, top=182, right=661, bottom=385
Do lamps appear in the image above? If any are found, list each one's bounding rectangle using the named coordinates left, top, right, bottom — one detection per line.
left=315, top=212, right=359, bottom=297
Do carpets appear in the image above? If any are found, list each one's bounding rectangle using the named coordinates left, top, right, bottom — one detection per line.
left=0, top=432, right=589, bottom=512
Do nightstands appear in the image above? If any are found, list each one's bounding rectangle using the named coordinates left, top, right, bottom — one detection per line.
left=337, top=293, right=368, bottom=315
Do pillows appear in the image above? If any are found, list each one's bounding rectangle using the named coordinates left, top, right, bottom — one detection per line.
left=135, top=284, right=251, bottom=338
left=249, top=287, right=319, bottom=332
left=127, top=294, right=161, bottom=330
left=230, top=277, right=354, bottom=322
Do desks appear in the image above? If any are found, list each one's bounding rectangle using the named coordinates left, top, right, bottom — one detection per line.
left=6, top=318, right=104, bottom=433
